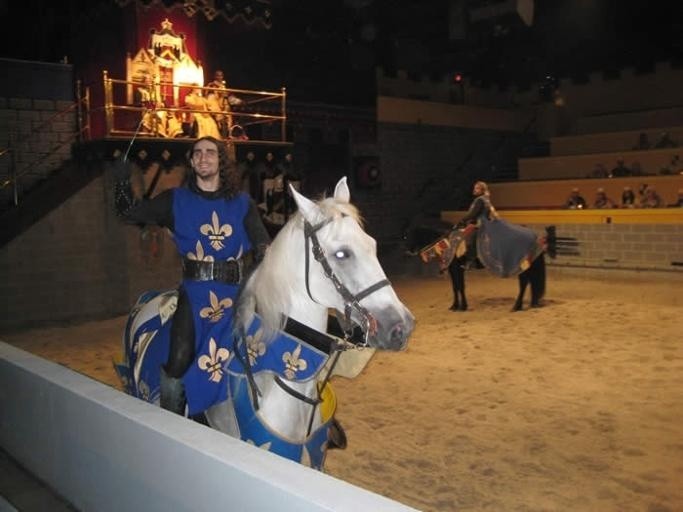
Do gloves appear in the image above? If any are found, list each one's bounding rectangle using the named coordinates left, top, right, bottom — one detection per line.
left=110, top=150, right=131, bottom=178
left=251, top=244, right=268, bottom=262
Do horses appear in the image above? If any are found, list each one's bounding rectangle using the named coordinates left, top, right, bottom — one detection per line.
left=400, top=207, right=548, bottom=312
left=121, top=174, right=413, bottom=474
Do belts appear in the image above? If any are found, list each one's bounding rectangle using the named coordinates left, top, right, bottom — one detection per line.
left=186, top=260, right=242, bottom=282
left=470, top=221, right=476, bottom=225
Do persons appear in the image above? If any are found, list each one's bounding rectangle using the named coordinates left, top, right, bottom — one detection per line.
left=459, top=181, right=493, bottom=271
left=589, top=188, right=614, bottom=209
left=639, top=187, right=664, bottom=208
left=281, top=152, right=302, bottom=181
left=633, top=133, right=652, bottom=150
left=666, top=189, right=683, bottom=208
left=135, top=76, right=184, bottom=138
left=661, top=154, right=683, bottom=175
left=204, top=69, right=246, bottom=138
left=237, top=151, right=256, bottom=196
left=157, top=146, right=179, bottom=169
left=617, top=187, right=636, bottom=209
left=261, top=161, right=281, bottom=180
left=656, top=132, right=677, bottom=149
left=183, top=83, right=223, bottom=140
left=563, top=186, right=587, bottom=209
left=113, top=136, right=274, bottom=418
left=184, top=149, right=192, bottom=159
left=609, top=159, right=632, bottom=177
left=257, top=151, right=282, bottom=200
left=133, top=146, right=153, bottom=172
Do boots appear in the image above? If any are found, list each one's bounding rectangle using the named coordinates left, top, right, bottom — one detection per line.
left=156, top=363, right=186, bottom=416
left=459, top=240, right=480, bottom=271
